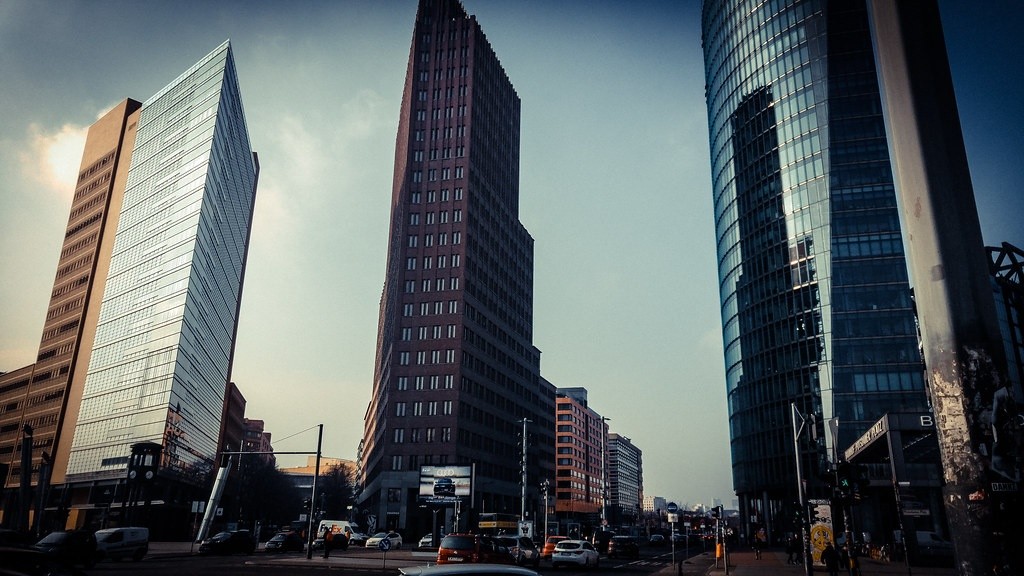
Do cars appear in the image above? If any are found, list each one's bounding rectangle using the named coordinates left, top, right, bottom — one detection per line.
left=436, top=532, right=518, bottom=566
left=264, top=532, right=304, bottom=554
left=492, top=534, right=540, bottom=568
left=365, top=531, right=403, bottom=550
left=198, top=531, right=256, bottom=557
left=541, top=536, right=572, bottom=561
left=552, top=540, right=600, bottom=571
left=417, top=533, right=446, bottom=549
left=670, top=534, right=712, bottom=548
left=648, top=534, right=666, bottom=549
left=433, top=478, right=456, bottom=497
left=397, top=563, right=543, bottom=576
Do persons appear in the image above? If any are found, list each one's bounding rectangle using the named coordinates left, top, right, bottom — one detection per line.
left=600, top=532, right=608, bottom=553
left=321, top=527, right=331, bottom=558
left=752, top=538, right=761, bottom=560
left=523, top=542, right=531, bottom=550
left=736, top=537, right=744, bottom=548
left=784, top=533, right=803, bottom=565
left=821, top=542, right=850, bottom=576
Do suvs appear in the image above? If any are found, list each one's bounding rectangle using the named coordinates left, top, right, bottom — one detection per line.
left=607, top=536, right=640, bottom=562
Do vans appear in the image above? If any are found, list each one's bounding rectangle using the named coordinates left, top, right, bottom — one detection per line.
left=275, top=519, right=369, bottom=552
left=0, top=525, right=150, bottom=576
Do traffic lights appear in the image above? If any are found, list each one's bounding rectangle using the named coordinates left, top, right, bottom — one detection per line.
left=711, top=506, right=722, bottom=518
left=839, top=462, right=849, bottom=491
left=807, top=502, right=820, bottom=525
